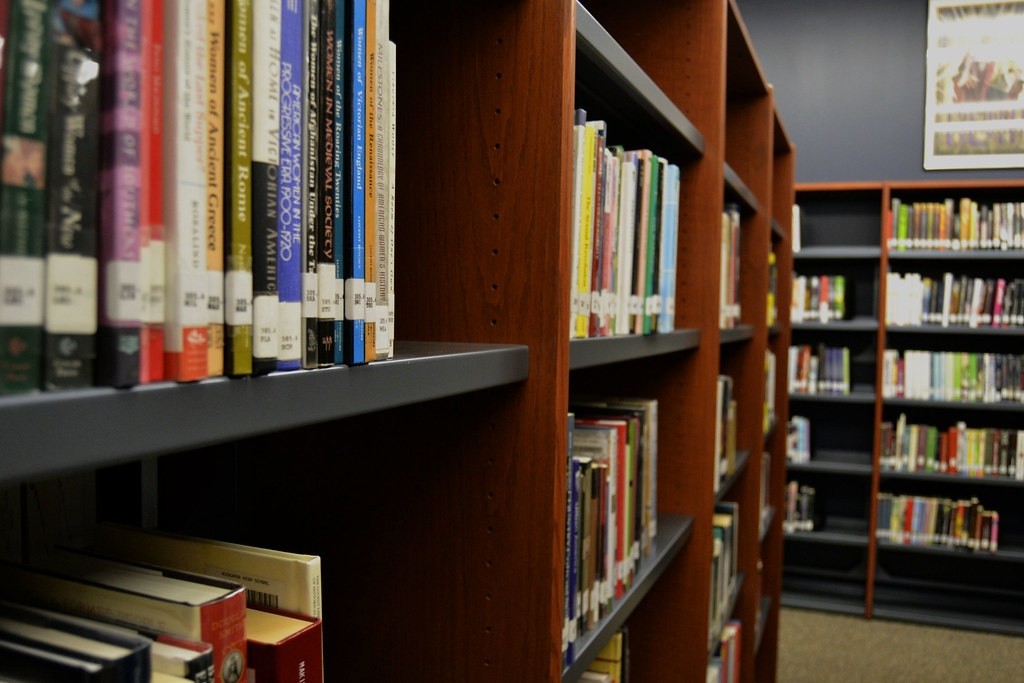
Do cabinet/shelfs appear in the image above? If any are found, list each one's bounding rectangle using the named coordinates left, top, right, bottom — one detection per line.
left=0, top=0, right=797, bottom=683
left=780, top=182, right=1024, bottom=638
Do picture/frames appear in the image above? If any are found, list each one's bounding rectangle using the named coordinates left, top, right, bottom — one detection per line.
left=922, top=0, right=1024, bottom=171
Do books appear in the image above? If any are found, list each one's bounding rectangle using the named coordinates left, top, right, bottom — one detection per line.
left=0, top=1, right=1024, bottom=682
